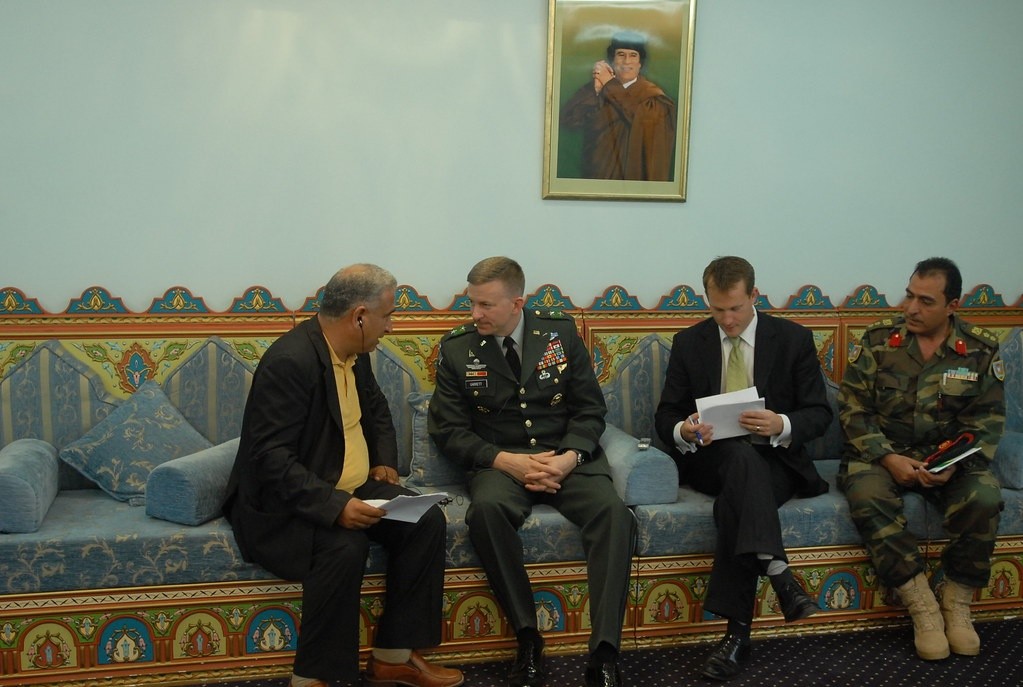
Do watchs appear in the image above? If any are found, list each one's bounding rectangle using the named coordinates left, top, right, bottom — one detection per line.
left=562, top=449, right=585, bottom=466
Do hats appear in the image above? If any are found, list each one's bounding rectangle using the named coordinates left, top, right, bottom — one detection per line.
left=607, top=33, right=646, bottom=62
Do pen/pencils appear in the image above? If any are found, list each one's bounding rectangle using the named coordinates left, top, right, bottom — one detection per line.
left=689, top=416, right=703, bottom=445
left=943, top=371, right=948, bottom=385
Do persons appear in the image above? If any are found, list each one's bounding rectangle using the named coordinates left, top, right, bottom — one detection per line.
left=227, top=264, right=465, bottom=687
left=427, top=257, right=639, bottom=687
left=838, top=257, right=1006, bottom=661
left=653, top=256, right=835, bottom=680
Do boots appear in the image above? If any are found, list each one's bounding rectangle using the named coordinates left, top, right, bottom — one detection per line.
left=933, top=571, right=981, bottom=656
left=890, top=571, right=950, bottom=660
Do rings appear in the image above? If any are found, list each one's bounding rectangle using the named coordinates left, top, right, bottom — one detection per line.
left=756, top=425, right=761, bottom=431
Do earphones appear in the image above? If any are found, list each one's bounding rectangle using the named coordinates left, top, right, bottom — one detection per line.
left=357, top=316, right=363, bottom=327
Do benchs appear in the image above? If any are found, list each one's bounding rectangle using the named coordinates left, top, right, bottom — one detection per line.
left=0, top=286, right=1023, bottom=687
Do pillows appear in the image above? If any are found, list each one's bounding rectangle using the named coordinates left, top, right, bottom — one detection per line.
left=405, top=392, right=464, bottom=488
left=60, top=377, right=215, bottom=505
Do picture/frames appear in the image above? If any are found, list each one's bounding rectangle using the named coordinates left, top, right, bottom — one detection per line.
left=542, top=0, right=697, bottom=203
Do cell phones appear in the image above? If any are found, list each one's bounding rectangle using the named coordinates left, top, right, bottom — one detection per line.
left=638, top=438, right=651, bottom=451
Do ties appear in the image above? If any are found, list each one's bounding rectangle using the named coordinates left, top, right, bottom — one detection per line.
left=503, top=336, right=521, bottom=384
left=724, top=336, right=749, bottom=393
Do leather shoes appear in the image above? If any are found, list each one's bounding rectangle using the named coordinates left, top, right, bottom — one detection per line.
left=586, top=662, right=621, bottom=687
left=287, top=679, right=329, bottom=687
left=363, top=648, right=464, bottom=687
left=508, top=636, right=545, bottom=687
left=699, top=634, right=751, bottom=679
left=777, top=580, right=811, bottom=622
